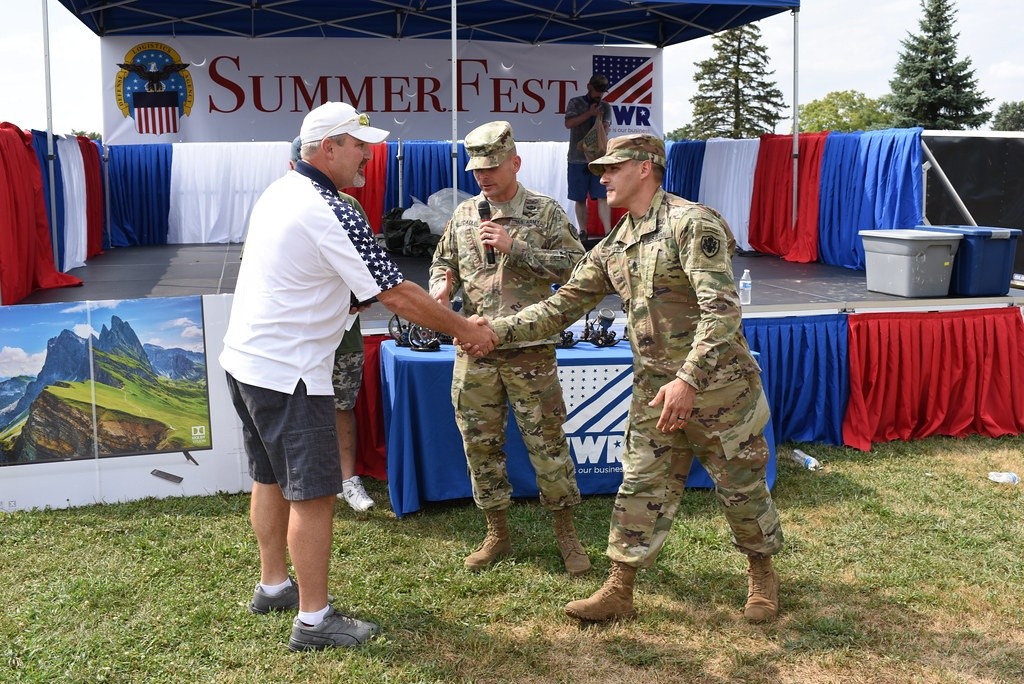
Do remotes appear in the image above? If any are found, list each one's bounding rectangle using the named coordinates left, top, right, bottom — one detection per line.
left=151, top=469, right=184, bottom=484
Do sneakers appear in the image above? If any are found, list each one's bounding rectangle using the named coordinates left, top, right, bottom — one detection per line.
left=336, top=476, right=375, bottom=513
left=289, top=602, right=377, bottom=653
left=248, top=575, right=336, bottom=616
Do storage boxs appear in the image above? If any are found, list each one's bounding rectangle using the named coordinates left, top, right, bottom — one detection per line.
left=859, top=229, right=963, bottom=297
left=915, top=225, right=1023, bottom=297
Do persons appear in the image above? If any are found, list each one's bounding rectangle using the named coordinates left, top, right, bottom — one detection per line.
left=564, top=73, right=617, bottom=241
left=427, top=118, right=592, bottom=575
left=453, top=132, right=785, bottom=623
left=219, top=99, right=501, bottom=649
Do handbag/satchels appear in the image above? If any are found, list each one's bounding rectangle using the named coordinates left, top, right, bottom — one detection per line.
left=577, top=113, right=606, bottom=162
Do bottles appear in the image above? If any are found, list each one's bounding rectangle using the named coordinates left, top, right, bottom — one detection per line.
left=739, top=268, right=751, bottom=305
left=791, top=448, right=824, bottom=473
left=988, top=471, right=1021, bottom=485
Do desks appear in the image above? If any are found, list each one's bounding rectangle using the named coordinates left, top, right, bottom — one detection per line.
left=378, top=340, right=777, bottom=518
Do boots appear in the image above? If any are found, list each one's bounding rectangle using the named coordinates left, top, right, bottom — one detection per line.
left=564, top=560, right=636, bottom=621
left=552, top=507, right=591, bottom=576
left=464, top=507, right=513, bottom=569
left=743, top=553, right=779, bottom=621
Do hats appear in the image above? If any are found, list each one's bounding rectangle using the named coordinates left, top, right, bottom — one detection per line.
left=300, top=101, right=390, bottom=144
left=589, top=74, right=608, bottom=92
left=464, top=121, right=515, bottom=171
left=587, top=134, right=667, bottom=177
left=290, top=136, right=302, bottom=161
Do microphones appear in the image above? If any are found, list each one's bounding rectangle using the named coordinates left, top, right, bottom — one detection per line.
left=477, top=201, right=496, bottom=264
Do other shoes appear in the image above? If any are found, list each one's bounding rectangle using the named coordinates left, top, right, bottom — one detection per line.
left=579, top=230, right=588, bottom=241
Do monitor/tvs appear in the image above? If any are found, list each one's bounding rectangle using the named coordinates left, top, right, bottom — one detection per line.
left=0, top=295, right=213, bottom=467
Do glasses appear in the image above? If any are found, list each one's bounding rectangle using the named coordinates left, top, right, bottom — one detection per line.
left=321, top=113, right=370, bottom=140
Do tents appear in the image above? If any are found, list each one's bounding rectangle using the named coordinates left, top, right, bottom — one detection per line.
left=37, top=0, right=802, bottom=269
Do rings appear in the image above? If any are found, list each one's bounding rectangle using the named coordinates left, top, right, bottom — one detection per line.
left=678, top=416, right=685, bottom=420
left=678, top=416, right=686, bottom=419
left=678, top=417, right=685, bottom=420
